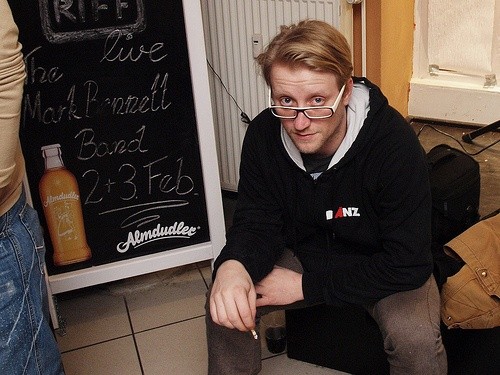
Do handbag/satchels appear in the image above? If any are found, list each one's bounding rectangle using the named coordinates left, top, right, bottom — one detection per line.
left=438, top=212, right=500, bottom=330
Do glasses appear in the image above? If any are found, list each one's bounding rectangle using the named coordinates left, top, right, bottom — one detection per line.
left=267, top=84, right=347, bottom=119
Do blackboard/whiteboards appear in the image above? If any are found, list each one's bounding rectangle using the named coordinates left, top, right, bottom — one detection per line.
left=7, top=0, right=227, bottom=296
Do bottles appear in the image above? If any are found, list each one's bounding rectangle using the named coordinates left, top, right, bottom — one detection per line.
left=38, top=143, right=92, bottom=266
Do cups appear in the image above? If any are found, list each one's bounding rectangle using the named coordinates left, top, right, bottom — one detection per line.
left=261, top=310, right=286, bottom=353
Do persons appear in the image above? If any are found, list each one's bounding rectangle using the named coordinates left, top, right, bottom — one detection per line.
left=203, top=19, right=453, bottom=374
left=0, top=1, right=66, bottom=375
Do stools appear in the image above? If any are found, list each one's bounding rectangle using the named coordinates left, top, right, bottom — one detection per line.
left=285, top=227, right=500, bottom=375
